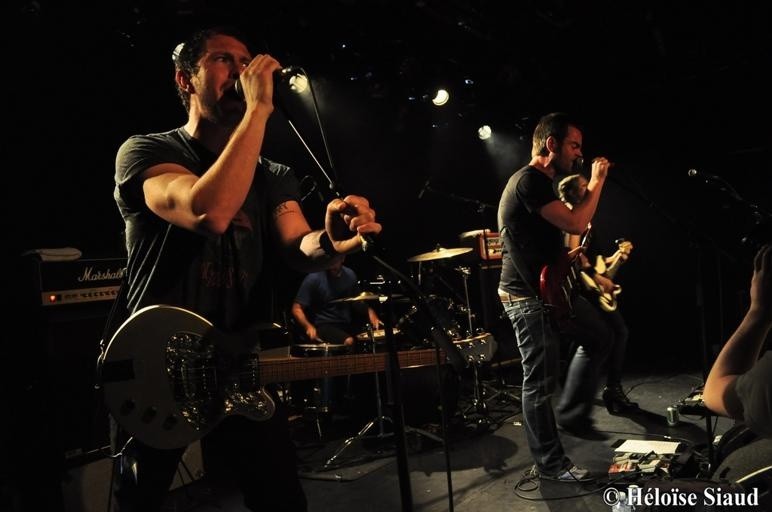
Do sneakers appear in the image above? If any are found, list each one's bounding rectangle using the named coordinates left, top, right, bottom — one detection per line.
left=527, top=462, right=602, bottom=483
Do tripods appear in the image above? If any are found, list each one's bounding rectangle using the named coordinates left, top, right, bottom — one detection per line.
left=464, top=279, right=523, bottom=415
left=326, top=324, right=444, bottom=466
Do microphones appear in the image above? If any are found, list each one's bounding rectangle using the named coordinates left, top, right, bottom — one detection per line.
left=235, top=65, right=300, bottom=97
left=688, top=169, right=703, bottom=186
left=571, top=155, right=617, bottom=174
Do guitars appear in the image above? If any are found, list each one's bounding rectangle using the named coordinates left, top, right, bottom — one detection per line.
left=580, top=238, right=633, bottom=313
left=100, top=304, right=497, bottom=450
left=539, top=222, right=593, bottom=319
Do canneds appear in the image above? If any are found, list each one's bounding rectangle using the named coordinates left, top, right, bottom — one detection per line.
left=667, top=406, right=679, bottom=426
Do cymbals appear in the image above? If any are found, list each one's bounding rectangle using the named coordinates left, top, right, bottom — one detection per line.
left=330, top=291, right=402, bottom=303
left=407, top=248, right=474, bottom=262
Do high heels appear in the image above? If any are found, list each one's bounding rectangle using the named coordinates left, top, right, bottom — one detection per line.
left=602, top=386, right=640, bottom=416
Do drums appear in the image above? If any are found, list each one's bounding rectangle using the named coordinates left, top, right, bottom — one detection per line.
left=356, top=328, right=403, bottom=354
left=293, top=343, right=347, bottom=411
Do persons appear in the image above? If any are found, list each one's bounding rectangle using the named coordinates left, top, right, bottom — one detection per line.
left=555, top=173, right=643, bottom=414
left=699, top=241, right=771, bottom=442
left=495, top=113, right=618, bottom=482
left=289, top=247, right=383, bottom=347
left=96, top=24, right=383, bottom=511
